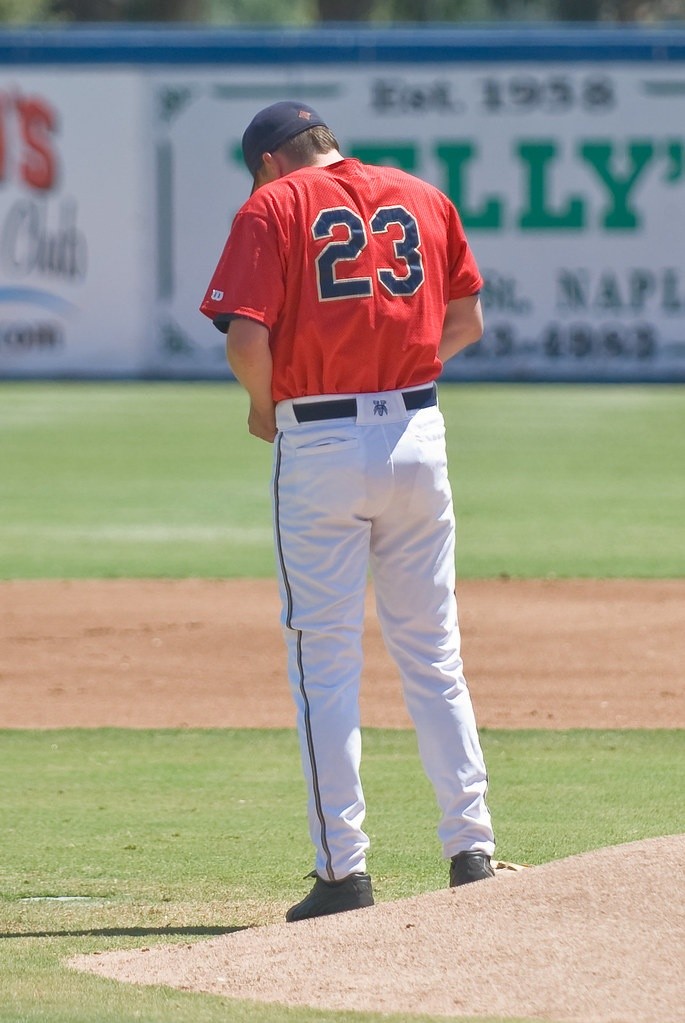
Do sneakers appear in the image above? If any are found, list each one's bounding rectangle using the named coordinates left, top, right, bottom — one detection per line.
left=450, top=850, right=496, bottom=888
left=286, top=870, right=375, bottom=923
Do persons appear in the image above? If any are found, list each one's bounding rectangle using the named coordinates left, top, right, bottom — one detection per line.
left=200, top=102, right=497, bottom=926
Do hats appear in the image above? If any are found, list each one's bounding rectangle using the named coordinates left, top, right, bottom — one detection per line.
left=242, top=100, right=328, bottom=198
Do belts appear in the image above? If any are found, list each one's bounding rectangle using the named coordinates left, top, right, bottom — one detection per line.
left=293, top=385, right=438, bottom=423
